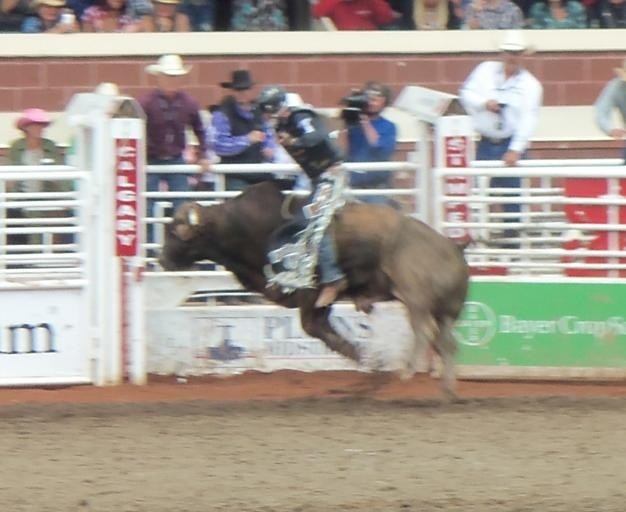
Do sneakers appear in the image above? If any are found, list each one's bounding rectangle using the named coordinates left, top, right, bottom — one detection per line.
left=313, top=277, right=349, bottom=309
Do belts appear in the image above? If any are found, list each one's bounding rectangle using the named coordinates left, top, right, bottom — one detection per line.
left=480, top=135, right=511, bottom=145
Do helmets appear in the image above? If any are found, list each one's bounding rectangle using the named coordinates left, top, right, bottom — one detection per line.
left=361, top=80, right=393, bottom=115
left=258, top=87, right=287, bottom=115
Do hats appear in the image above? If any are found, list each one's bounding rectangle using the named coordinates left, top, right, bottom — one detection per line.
left=282, top=93, right=309, bottom=109
left=30, top=0, right=67, bottom=9
left=492, top=31, right=538, bottom=55
left=16, top=108, right=53, bottom=129
left=219, top=68, right=256, bottom=90
left=145, top=54, right=192, bottom=78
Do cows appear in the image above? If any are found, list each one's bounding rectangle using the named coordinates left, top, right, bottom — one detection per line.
left=158, top=180, right=474, bottom=401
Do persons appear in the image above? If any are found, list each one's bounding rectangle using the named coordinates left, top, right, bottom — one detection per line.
left=335, top=76, right=399, bottom=209
left=205, top=67, right=280, bottom=205
left=593, top=59, right=626, bottom=162
left=458, top=30, right=544, bottom=263
left=3, top=107, right=77, bottom=271
left=255, top=82, right=350, bottom=311
left=1, top=0, right=625, bottom=34
left=140, top=54, right=210, bottom=269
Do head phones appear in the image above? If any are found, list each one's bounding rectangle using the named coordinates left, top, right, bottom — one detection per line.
left=364, top=78, right=391, bottom=106
